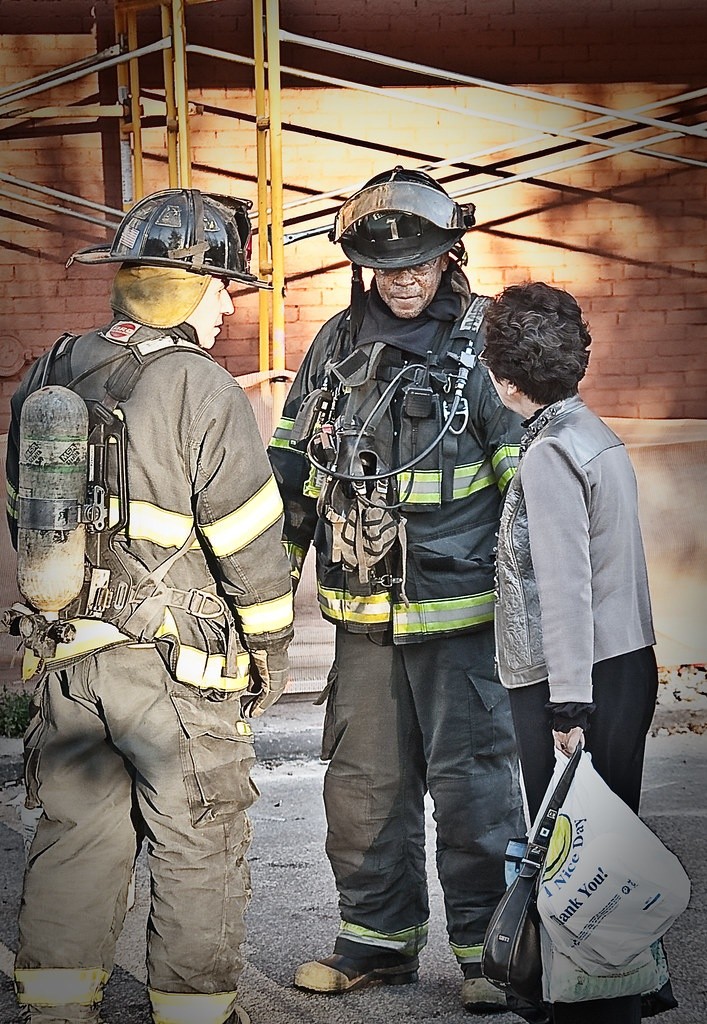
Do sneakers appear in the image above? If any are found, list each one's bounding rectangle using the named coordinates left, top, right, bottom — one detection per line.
left=222, top=1004, right=251, bottom=1024
left=294, top=953, right=420, bottom=993
left=461, top=964, right=510, bottom=1014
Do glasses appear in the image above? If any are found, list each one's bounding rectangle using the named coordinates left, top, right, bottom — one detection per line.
left=478, top=350, right=491, bottom=369
left=373, top=258, right=438, bottom=276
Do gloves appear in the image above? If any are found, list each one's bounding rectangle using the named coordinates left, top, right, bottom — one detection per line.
left=240, top=625, right=294, bottom=720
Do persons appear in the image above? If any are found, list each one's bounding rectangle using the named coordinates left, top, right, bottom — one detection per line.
left=475, top=281, right=658, bottom=828
left=0, top=187, right=295, bottom=1024
left=265, top=166, right=529, bottom=1012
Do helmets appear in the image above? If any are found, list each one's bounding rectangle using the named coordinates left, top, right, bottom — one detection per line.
left=65, top=188, right=276, bottom=291
left=329, top=166, right=475, bottom=268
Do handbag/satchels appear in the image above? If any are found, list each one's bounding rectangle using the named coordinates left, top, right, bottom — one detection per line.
left=480, top=740, right=691, bottom=1024
